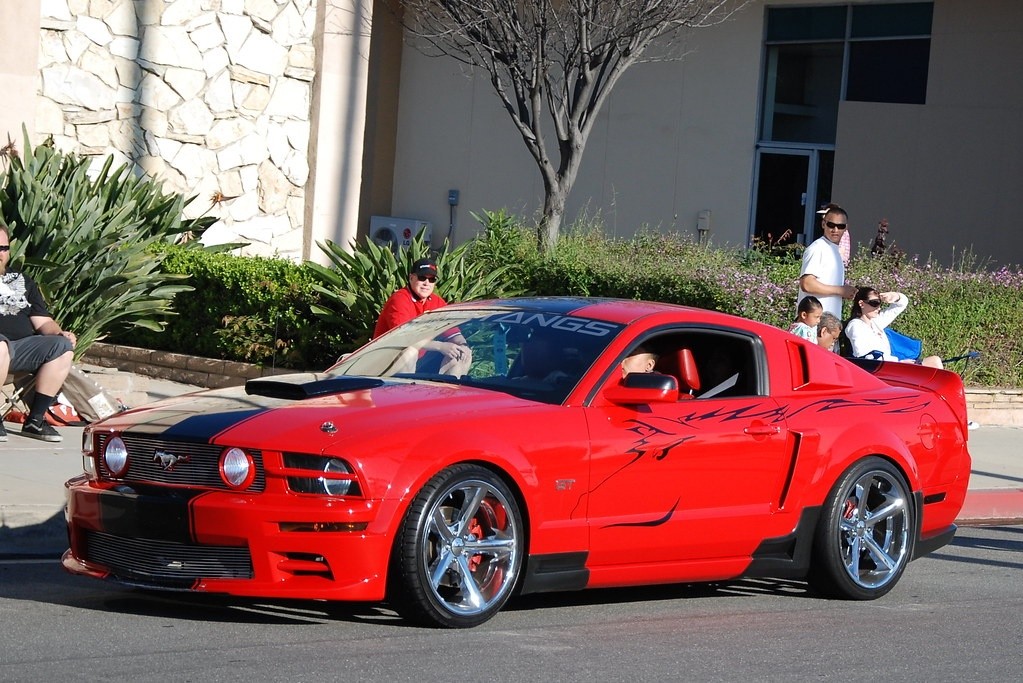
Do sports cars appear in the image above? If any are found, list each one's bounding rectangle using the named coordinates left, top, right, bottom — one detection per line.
left=60, top=297, right=972, bottom=628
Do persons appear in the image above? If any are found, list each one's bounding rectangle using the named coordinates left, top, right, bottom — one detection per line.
left=785, top=202, right=945, bottom=370
left=0, top=223, right=77, bottom=443
left=372, top=257, right=473, bottom=380
left=541, top=339, right=663, bottom=395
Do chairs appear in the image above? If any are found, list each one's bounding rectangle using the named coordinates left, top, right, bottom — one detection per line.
left=650, top=332, right=701, bottom=399
left=516, top=343, right=562, bottom=381
left=838, top=320, right=922, bottom=365
left=0, top=371, right=38, bottom=425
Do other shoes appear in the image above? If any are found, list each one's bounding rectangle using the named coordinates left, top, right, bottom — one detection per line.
left=968, top=420, right=979, bottom=430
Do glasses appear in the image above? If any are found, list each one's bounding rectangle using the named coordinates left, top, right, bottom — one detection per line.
left=413, top=274, right=436, bottom=283
left=863, top=298, right=881, bottom=307
left=824, top=218, right=847, bottom=230
left=823, top=326, right=839, bottom=343
left=0, top=245, right=10, bottom=252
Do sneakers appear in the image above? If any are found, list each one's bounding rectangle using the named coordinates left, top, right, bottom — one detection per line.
left=0, top=416, right=8, bottom=441
left=21, top=416, right=63, bottom=441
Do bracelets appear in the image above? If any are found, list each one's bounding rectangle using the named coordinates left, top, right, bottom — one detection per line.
left=458, top=343, right=471, bottom=350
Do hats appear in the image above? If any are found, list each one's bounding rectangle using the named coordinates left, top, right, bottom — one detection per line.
left=411, top=259, right=436, bottom=276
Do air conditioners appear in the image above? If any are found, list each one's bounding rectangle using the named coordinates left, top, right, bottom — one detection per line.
left=370, top=216, right=432, bottom=256
